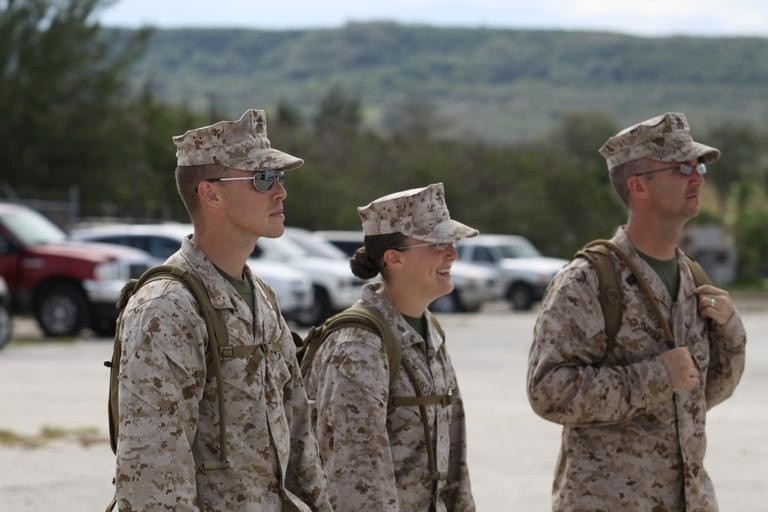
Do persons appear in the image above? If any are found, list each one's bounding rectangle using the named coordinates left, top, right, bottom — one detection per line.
left=108, top=107, right=337, bottom=512
left=523, top=110, right=750, bottom=511
left=295, top=180, right=481, bottom=511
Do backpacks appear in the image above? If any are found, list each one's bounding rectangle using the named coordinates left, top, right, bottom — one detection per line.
left=291, top=306, right=453, bottom=415
left=103, top=264, right=281, bottom=456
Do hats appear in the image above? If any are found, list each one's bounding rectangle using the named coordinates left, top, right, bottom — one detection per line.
left=172, top=108, right=305, bottom=175
left=357, top=181, right=478, bottom=243
left=598, top=111, right=721, bottom=171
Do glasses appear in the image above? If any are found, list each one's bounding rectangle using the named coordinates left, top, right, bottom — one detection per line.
left=627, top=158, right=708, bottom=179
left=192, top=168, right=289, bottom=192
left=393, top=240, right=457, bottom=253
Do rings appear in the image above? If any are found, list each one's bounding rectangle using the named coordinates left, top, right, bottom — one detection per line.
left=709, top=296, right=717, bottom=308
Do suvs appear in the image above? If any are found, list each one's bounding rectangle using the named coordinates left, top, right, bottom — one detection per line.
left=456, top=233, right=572, bottom=312
left=0, top=202, right=156, bottom=337
